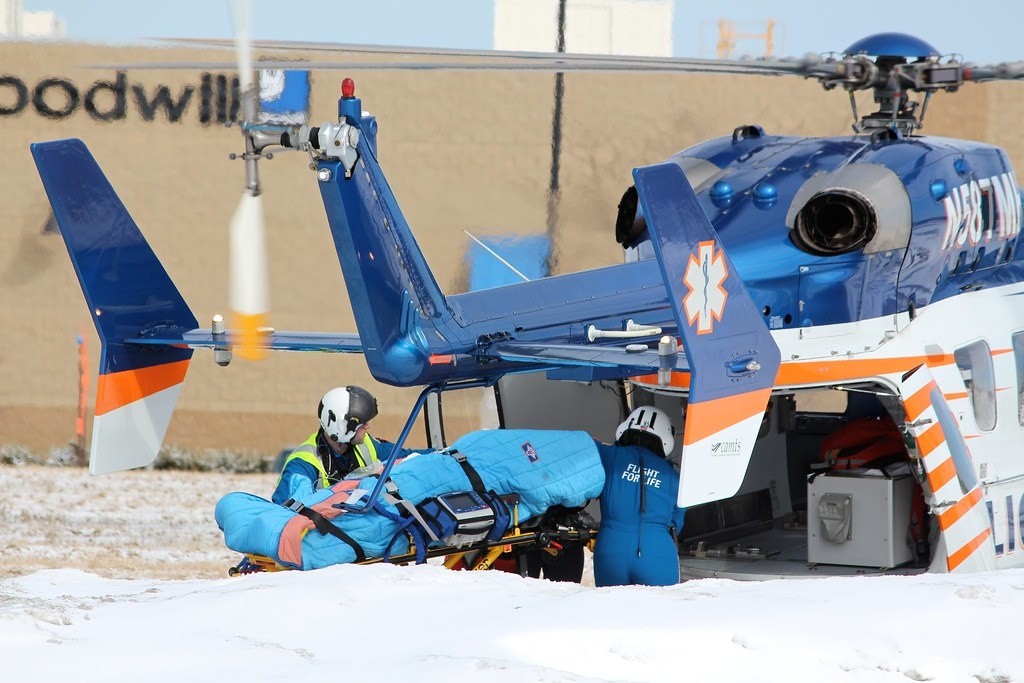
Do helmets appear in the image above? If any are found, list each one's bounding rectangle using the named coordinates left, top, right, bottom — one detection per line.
left=615, top=405, right=677, bottom=458
left=316, top=385, right=379, bottom=442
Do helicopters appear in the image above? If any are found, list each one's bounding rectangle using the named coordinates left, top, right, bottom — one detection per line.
left=27, top=30, right=1024, bottom=572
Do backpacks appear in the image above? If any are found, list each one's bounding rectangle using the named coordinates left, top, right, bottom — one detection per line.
left=808, top=406, right=909, bottom=483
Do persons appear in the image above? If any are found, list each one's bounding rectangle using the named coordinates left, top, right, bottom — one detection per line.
left=591, top=405, right=688, bottom=587
left=270, top=385, right=448, bottom=510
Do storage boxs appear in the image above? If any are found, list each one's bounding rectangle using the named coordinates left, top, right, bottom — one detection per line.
left=808, top=475, right=916, bottom=569
